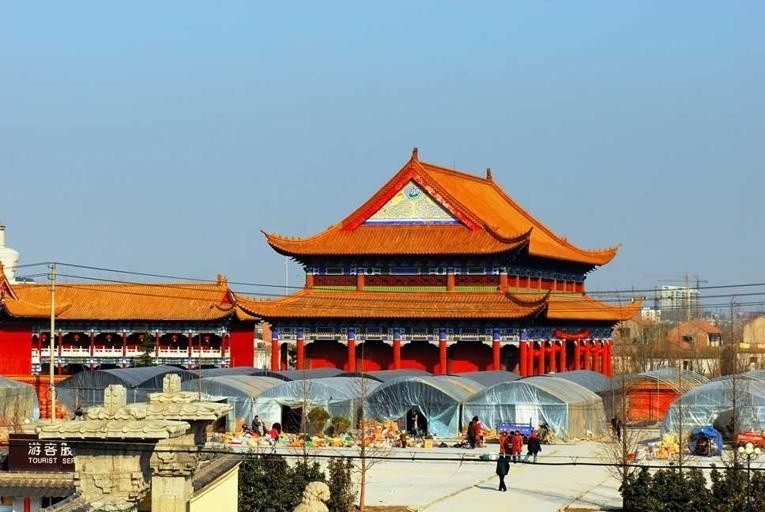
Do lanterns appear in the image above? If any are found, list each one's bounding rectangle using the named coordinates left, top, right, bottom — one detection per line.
left=106, top=334, right=112, bottom=342
left=138, top=334, right=145, bottom=341
left=72, top=333, right=80, bottom=342
left=171, top=335, right=178, bottom=342
left=41, top=334, right=47, bottom=342
left=204, top=335, right=212, bottom=342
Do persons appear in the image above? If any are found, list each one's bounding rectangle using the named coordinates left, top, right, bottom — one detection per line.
left=499, top=430, right=509, bottom=460
left=496, top=452, right=510, bottom=491
left=505, top=431, right=515, bottom=462
left=522, top=430, right=543, bottom=463
left=251, top=414, right=263, bottom=438
left=610, top=413, right=623, bottom=442
left=511, top=430, right=524, bottom=463
left=269, top=423, right=283, bottom=444
left=411, top=409, right=421, bottom=438
left=472, top=415, right=483, bottom=447
left=467, top=420, right=479, bottom=449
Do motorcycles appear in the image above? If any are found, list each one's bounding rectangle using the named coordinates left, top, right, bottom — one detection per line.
left=242, top=419, right=270, bottom=437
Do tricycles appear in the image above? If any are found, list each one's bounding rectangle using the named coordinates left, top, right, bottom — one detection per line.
left=497, top=417, right=556, bottom=444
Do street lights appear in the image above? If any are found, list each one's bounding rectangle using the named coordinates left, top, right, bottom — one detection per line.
left=738, top=443, right=760, bottom=507
